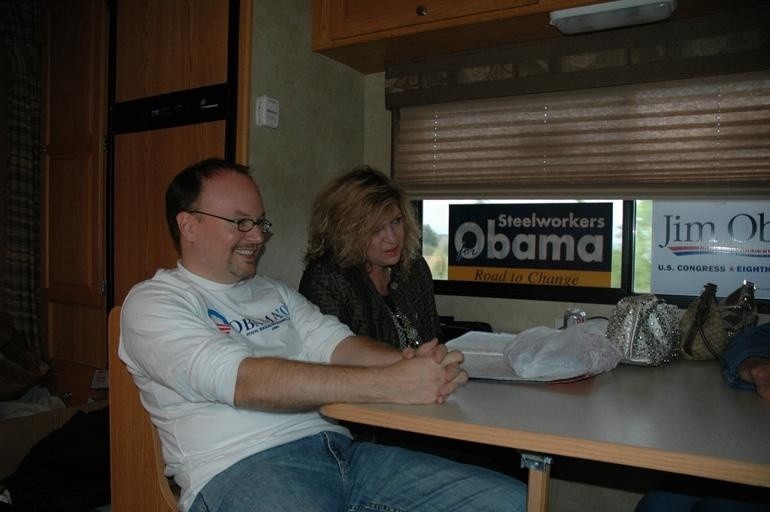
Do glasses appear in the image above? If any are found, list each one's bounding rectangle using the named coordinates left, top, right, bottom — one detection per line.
left=188, top=209, right=272, bottom=234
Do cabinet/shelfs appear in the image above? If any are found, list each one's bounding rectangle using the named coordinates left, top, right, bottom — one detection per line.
left=37, top=0, right=253, bottom=404
left=312, top=0, right=617, bottom=75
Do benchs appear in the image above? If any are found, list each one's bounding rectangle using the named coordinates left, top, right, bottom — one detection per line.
left=108, top=307, right=493, bottom=512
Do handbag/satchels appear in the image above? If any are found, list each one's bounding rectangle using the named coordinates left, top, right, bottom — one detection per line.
left=678, top=279, right=762, bottom=363
left=605, top=290, right=683, bottom=367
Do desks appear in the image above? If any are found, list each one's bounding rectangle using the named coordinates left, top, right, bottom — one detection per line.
left=319, top=360, right=769, bottom=511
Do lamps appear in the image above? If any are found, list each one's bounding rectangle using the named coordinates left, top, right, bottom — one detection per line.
left=550, top=0, right=677, bottom=36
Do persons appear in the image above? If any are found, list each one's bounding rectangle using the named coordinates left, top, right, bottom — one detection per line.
left=294, top=164, right=443, bottom=361
left=115, top=157, right=529, bottom=511
left=635, top=317, right=770, bottom=510
